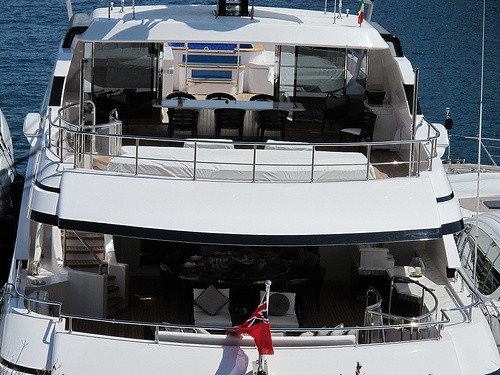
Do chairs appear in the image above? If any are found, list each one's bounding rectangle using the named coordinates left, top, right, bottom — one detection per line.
left=337, top=111, right=378, bottom=163
left=166, top=92, right=198, bottom=137
left=192, top=288, right=233, bottom=327
left=205, top=94, right=246, bottom=138
left=259, top=291, right=300, bottom=331
left=249, top=94, right=289, bottom=138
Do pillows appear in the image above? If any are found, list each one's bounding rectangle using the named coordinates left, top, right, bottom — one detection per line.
left=268, top=293, right=290, bottom=316
left=194, top=285, right=232, bottom=316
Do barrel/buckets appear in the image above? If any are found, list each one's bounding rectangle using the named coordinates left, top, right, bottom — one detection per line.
left=365, top=88, right=385, bottom=105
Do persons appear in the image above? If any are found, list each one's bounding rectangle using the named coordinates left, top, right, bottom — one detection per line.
left=279, top=247, right=304, bottom=265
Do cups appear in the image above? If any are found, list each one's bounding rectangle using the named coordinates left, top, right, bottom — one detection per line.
left=415, top=267, right=421, bottom=275
left=411, top=271, right=416, bottom=275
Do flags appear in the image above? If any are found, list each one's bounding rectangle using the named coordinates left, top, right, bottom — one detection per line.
left=358, top=0, right=364, bottom=24
left=227, top=302, right=273, bottom=355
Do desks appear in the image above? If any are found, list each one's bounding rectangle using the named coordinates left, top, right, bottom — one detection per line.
left=285, top=84, right=328, bottom=138
left=153, top=98, right=306, bottom=111
